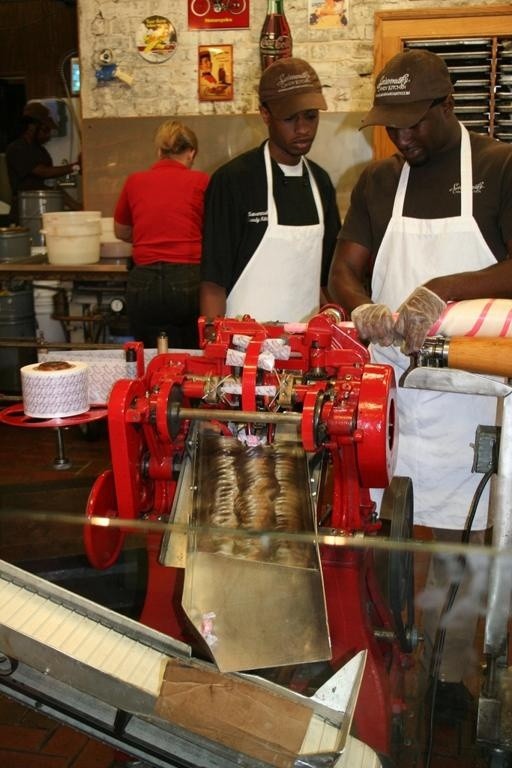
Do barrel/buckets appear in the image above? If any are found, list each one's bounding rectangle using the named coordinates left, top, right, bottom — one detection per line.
left=0, top=189, right=64, bottom=393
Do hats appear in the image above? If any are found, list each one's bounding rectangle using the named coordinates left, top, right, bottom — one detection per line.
left=256, top=57, right=327, bottom=120
left=358, top=47, right=455, bottom=132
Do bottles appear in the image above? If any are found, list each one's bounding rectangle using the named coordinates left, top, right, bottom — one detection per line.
left=259, top=0, right=292, bottom=70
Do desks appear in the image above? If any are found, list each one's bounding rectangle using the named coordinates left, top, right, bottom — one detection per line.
left=0, top=252, right=131, bottom=283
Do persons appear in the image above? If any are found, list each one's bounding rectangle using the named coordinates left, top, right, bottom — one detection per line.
left=324, top=45, right=511, bottom=727
left=201, top=52, right=227, bottom=94
left=112, top=120, right=211, bottom=351
left=196, top=56, right=341, bottom=328
left=5, top=102, right=80, bottom=228
